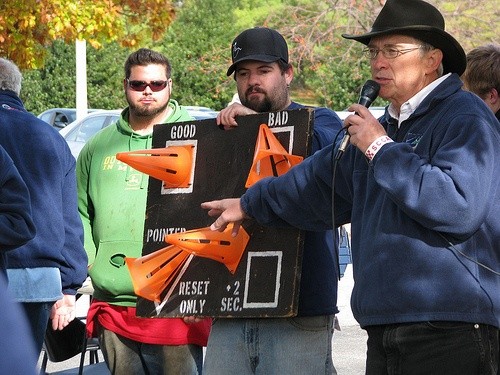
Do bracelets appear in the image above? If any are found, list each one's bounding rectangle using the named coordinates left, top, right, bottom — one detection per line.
left=365, top=136, right=394, bottom=161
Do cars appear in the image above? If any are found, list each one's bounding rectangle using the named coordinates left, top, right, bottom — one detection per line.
left=38, top=104, right=390, bottom=164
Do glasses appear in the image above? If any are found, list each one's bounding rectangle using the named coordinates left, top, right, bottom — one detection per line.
left=361, top=44, right=430, bottom=59
left=126, top=79, right=168, bottom=92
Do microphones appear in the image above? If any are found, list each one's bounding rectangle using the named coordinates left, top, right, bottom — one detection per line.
left=335, top=80, right=380, bottom=160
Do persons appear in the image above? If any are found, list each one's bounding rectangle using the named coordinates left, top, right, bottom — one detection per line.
left=0, top=58, right=88, bottom=375
left=75, top=48, right=213, bottom=375
left=201, top=27, right=345, bottom=375
left=201, top=0, right=500, bottom=375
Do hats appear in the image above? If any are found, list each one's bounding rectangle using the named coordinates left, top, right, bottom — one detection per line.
left=226, top=26, right=288, bottom=76
left=340, top=0, right=467, bottom=79
